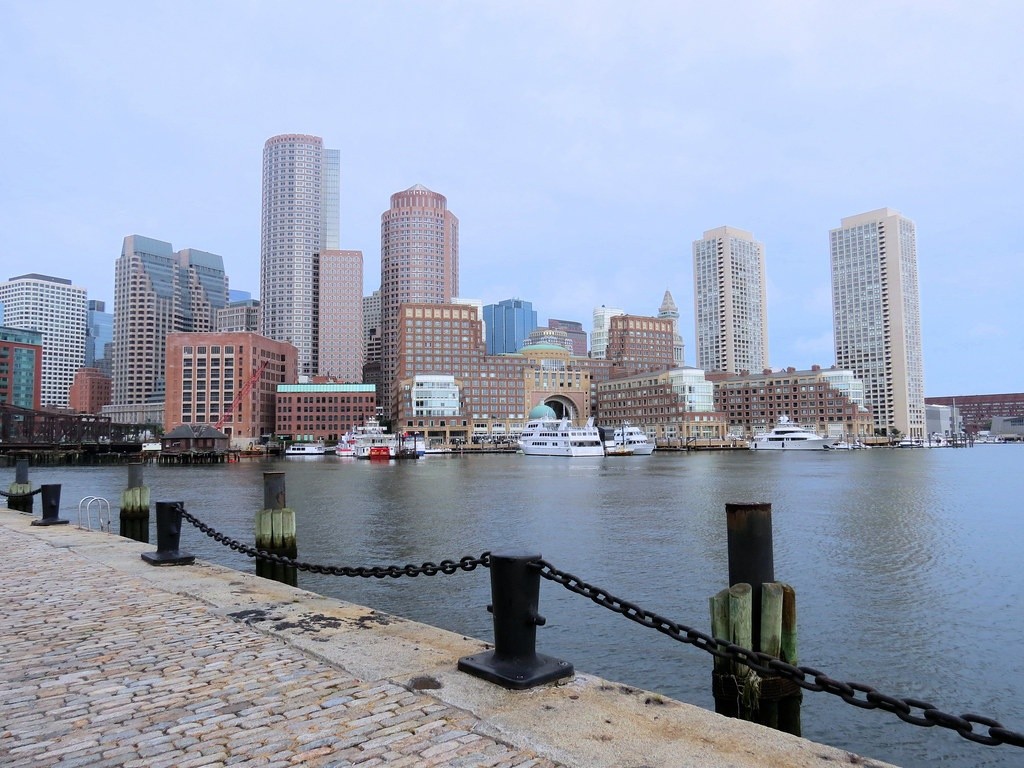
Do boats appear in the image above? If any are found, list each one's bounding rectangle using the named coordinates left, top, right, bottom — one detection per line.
left=519, top=414, right=606, bottom=457
left=614, top=427, right=657, bottom=455
left=899, top=433, right=1002, bottom=450
left=749, top=415, right=839, bottom=450
left=335, top=416, right=427, bottom=460
left=285, top=440, right=327, bottom=454
